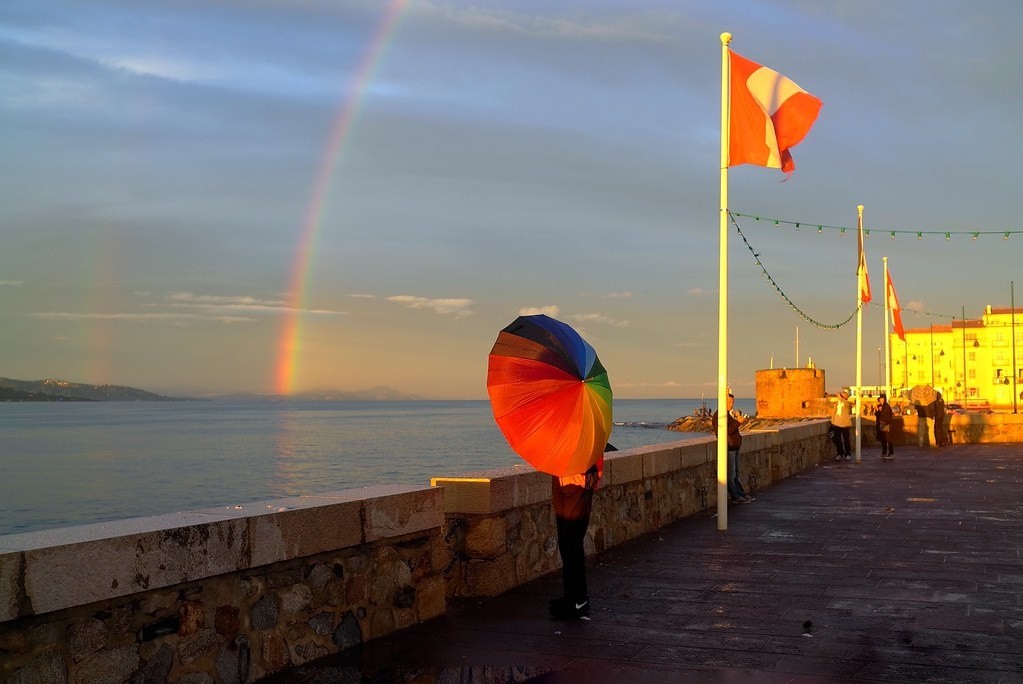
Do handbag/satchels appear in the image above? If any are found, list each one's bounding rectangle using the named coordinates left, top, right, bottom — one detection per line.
left=879, top=421, right=891, bottom=432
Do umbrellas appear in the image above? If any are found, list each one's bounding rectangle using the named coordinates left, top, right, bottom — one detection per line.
left=907, top=385, right=938, bottom=407
left=486, top=312, right=613, bottom=474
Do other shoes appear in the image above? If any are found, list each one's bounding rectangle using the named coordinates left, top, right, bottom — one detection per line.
left=733, top=497, right=750, bottom=503
left=549, top=596, right=590, bottom=622
left=880, top=455, right=886, bottom=458
left=884, top=455, right=895, bottom=459
left=744, top=495, right=757, bottom=501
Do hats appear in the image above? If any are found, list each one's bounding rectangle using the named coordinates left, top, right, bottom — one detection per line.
left=842, top=387, right=850, bottom=395
left=877, top=394, right=886, bottom=402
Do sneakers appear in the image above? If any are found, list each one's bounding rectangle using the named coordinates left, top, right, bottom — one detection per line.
left=846, top=455, right=851, bottom=460
left=835, top=455, right=843, bottom=460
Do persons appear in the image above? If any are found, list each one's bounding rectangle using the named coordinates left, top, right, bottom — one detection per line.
left=934, top=392, right=945, bottom=446
left=548, top=455, right=599, bottom=620
left=874, top=394, right=894, bottom=459
left=830, top=387, right=853, bottom=459
left=713, top=395, right=756, bottom=503
left=915, top=402, right=938, bottom=447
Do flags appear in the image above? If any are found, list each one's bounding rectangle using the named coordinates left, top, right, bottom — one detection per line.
left=856, top=214, right=872, bottom=306
left=886, top=271, right=906, bottom=341
left=726, top=52, right=823, bottom=173
